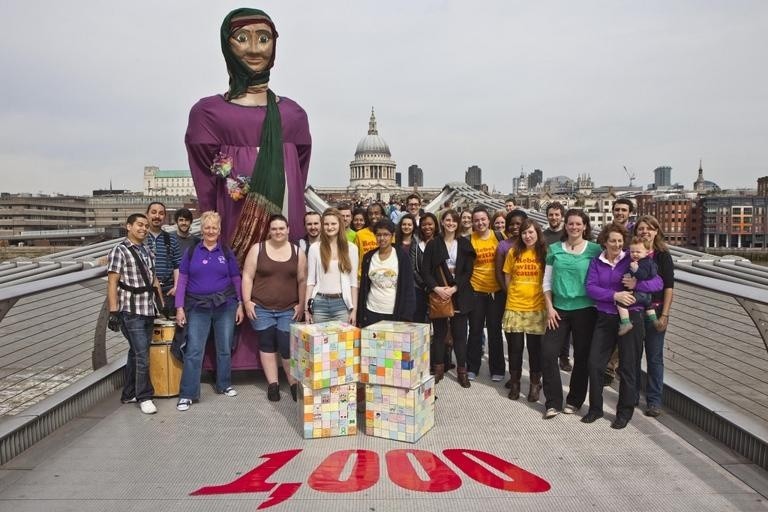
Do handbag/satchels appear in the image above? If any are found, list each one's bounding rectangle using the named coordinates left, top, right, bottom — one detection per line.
left=426, top=265, right=454, bottom=319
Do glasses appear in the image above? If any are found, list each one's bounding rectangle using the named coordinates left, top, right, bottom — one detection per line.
left=406, top=203, right=420, bottom=207
left=307, top=297, right=315, bottom=314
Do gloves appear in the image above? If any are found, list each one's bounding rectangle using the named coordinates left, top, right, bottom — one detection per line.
left=109, top=313, right=121, bottom=331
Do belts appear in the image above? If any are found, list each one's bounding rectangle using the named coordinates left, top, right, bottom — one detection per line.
left=313, top=293, right=343, bottom=299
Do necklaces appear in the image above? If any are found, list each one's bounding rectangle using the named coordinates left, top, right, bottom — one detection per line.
left=565, top=240, right=585, bottom=250
left=330, top=248, right=337, bottom=258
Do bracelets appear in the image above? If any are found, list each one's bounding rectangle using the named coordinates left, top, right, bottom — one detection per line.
left=660, top=312, right=669, bottom=317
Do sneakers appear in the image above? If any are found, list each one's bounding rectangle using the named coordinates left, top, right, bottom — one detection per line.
left=176, top=398, right=192, bottom=411
left=562, top=403, right=578, bottom=414
left=221, top=386, right=238, bottom=397
left=468, top=371, right=476, bottom=381
left=617, top=324, right=634, bottom=336
left=140, top=400, right=158, bottom=414
left=646, top=406, right=660, bottom=417
left=611, top=414, right=630, bottom=429
left=291, top=383, right=298, bottom=402
left=266, top=382, right=281, bottom=402
left=123, top=398, right=139, bottom=404
left=580, top=412, right=603, bottom=424
left=491, top=372, right=502, bottom=381
left=543, top=407, right=562, bottom=417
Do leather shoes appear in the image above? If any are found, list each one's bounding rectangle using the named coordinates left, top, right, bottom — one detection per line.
left=559, top=357, right=572, bottom=371
left=509, top=374, right=521, bottom=400
left=434, top=364, right=445, bottom=384
left=457, top=368, right=470, bottom=386
left=528, top=372, right=542, bottom=402
left=604, top=376, right=613, bottom=385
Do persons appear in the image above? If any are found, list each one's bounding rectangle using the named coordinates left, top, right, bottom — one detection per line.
left=357, top=204, right=395, bottom=289
left=299, top=211, right=322, bottom=255
left=184, top=8, right=312, bottom=379
left=241, top=214, right=307, bottom=402
left=492, top=211, right=509, bottom=231
left=542, top=209, right=604, bottom=419
left=633, top=216, right=675, bottom=417
left=356, top=219, right=416, bottom=328
left=596, top=200, right=637, bottom=248
left=107, top=214, right=164, bottom=413
left=304, top=208, right=359, bottom=326
left=385, top=201, right=407, bottom=224
left=462, top=207, right=509, bottom=381
left=338, top=204, right=356, bottom=243
left=351, top=213, right=365, bottom=231
left=459, top=209, right=475, bottom=235
left=503, top=218, right=549, bottom=401
left=419, top=209, right=477, bottom=388
left=145, top=202, right=183, bottom=319
left=177, top=211, right=243, bottom=410
left=505, top=199, right=516, bottom=213
left=409, top=213, right=442, bottom=323
left=168, top=208, right=201, bottom=253
left=617, top=235, right=664, bottom=335
left=396, top=214, right=420, bottom=321
left=406, top=194, right=423, bottom=228
left=581, top=223, right=664, bottom=428
left=494, top=210, right=528, bottom=388
left=541, top=202, right=568, bottom=243
